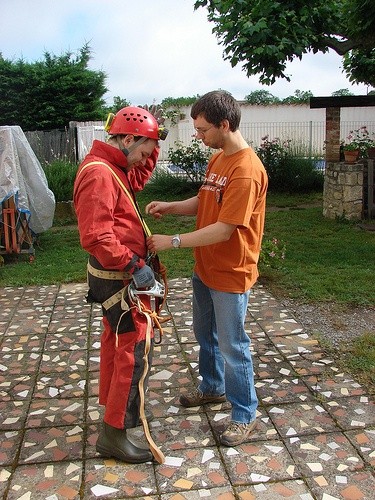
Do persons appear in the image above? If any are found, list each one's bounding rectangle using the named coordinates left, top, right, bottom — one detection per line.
left=73, top=106, right=159, bottom=462
left=146, top=91, right=268, bottom=446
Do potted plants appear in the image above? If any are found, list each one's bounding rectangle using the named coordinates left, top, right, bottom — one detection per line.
left=344, top=151, right=359, bottom=163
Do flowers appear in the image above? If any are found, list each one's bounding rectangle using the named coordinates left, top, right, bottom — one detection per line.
left=340, top=126, right=375, bottom=151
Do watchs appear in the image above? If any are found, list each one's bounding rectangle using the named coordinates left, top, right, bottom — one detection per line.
left=171, top=233, right=180, bottom=248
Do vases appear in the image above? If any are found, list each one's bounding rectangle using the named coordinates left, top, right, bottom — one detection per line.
left=367, top=148, right=375, bottom=159
